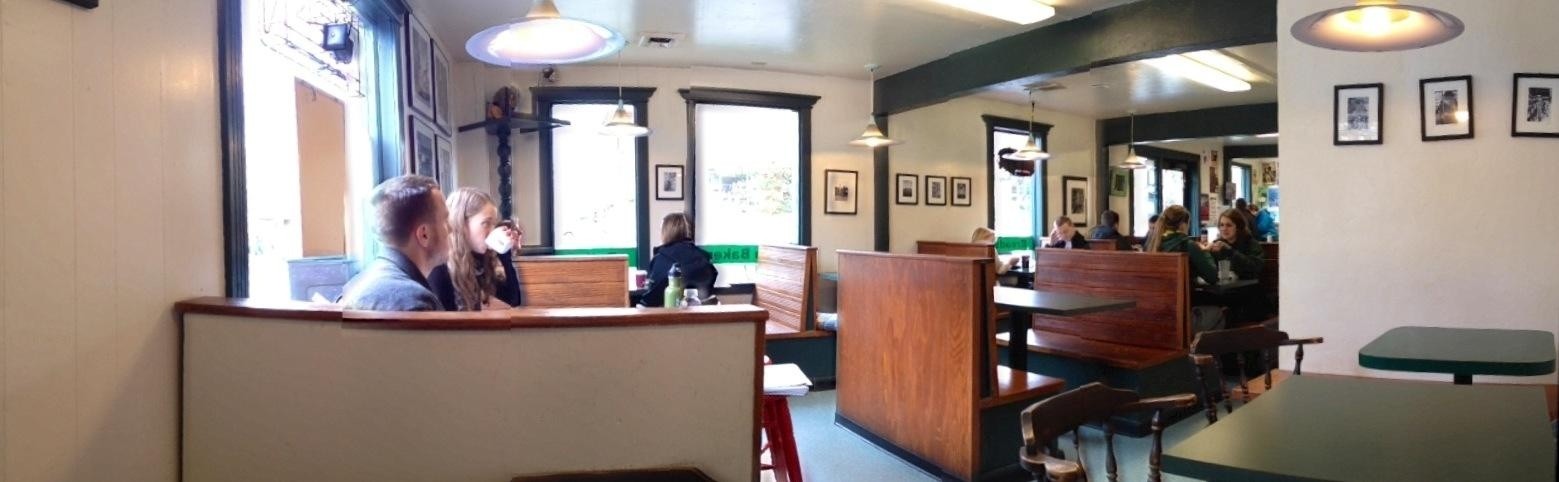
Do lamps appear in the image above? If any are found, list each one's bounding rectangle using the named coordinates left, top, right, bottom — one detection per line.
left=461, top=3, right=626, bottom=73
left=321, top=23, right=349, bottom=52
left=1002, top=100, right=1054, bottom=162
left=1117, top=115, right=1147, bottom=164
left=847, top=63, right=904, bottom=150
left=1288, top=0, right=1465, bottom=53
left=602, top=34, right=652, bottom=143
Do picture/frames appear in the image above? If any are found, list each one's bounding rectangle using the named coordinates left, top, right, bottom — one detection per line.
left=924, top=175, right=948, bottom=207
left=1331, top=82, right=1384, bottom=146
left=1509, top=72, right=1559, bottom=139
left=951, top=176, right=973, bottom=207
left=895, top=173, right=920, bottom=206
left=1062, top=174, right=1088, bottom=227
left=653, top=163, right=683, bottom=200
left=1416, top=74, right=1475, bottom=140
left=1108, top=166, right=1130, bottom=197
left=822, top=168, right=860, bottom=215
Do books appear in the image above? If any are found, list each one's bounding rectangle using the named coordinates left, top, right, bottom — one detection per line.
left=762, top=363, right=812, bottom=396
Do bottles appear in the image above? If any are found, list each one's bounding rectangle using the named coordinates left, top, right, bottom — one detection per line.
left=680, top=289, right=701, bottom=308
left=663, top=263, right=685, bottom=307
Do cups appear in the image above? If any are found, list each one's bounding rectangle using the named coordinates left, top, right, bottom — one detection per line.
left=1218, top=260, right=1230, bottom=279
left=636, top=271, right=646, bottom=287
left=1022, top=256, right=1030, bottom=268
left=485, top=226, right=514, bottom=255
left=1267, top=235, right=1272, bottom=243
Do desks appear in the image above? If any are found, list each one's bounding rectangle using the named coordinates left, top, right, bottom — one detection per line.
left=1356, top=325, right=1558, bottom=386
left=1156, top=371, right=1558, bottom=482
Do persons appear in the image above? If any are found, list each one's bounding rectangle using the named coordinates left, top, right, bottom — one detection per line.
left=430, top=187, right=524, bottom=311
left=1349, top=98, right=1357, bottom=128
left=335, top=173, right=457, bottom=312
left=1141, top=196, right=1279, bottom=402
left=640, top=213, right=718, bottom=306
left=971, top=228, right=1019, bottom=286
left=1093, top=209, right=1132, bottom=250
left=1044, top=216, right=1090, bottom=249
left=1356, top=101, right=1367, bottom=121
